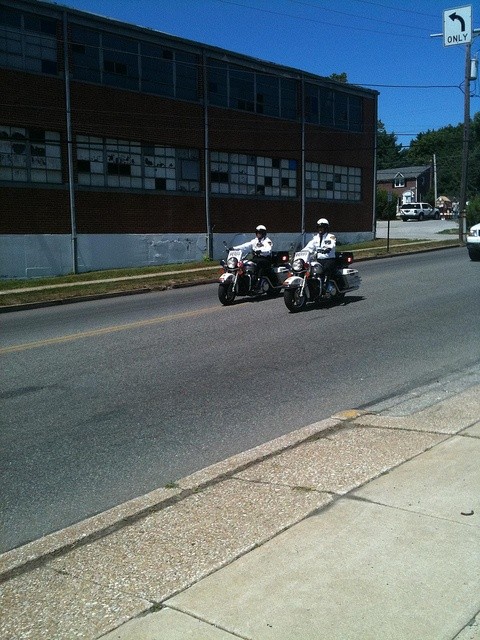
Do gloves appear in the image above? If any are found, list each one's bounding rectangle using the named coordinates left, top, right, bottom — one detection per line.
left=226, top=247, right=234, bottom=253
left=322, top=248, right=330, bottom=254
left=255, top=249, right=261, bottom=256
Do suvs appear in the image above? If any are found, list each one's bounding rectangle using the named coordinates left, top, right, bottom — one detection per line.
left=400, top=201, right=441, bottom=221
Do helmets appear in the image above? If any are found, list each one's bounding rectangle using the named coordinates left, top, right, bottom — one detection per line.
left=255, top=225, right=266, bottom=236
left=316, top=218, right=329, bottom=231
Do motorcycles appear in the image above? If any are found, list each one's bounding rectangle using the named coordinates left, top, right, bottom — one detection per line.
left=216, top=232, right=293, bottom=305
left=281, top=231, right=362, bottom=313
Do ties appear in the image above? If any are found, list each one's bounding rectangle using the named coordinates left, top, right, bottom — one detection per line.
left=319, top=236, right=322, bottom=248
left=258, top=239, right=261, bottom=243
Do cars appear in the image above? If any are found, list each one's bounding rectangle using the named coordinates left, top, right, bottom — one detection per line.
left=466, top=223, right=480, bottom=261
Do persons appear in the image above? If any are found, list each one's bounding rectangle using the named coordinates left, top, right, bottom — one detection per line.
left=226, top=225, right=273, bottom=296
left=300, top=218, right=337, bottom=293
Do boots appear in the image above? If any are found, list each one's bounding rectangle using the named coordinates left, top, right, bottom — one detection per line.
left=321, top=276, right=328, bottom=295
left=250, top=278, right=260, bottom=296
